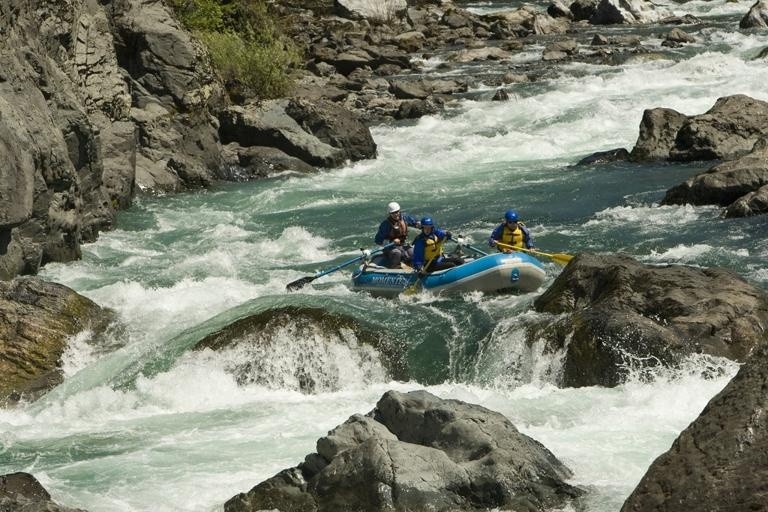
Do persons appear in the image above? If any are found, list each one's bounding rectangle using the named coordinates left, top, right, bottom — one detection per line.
left=375, top=202, right=421, bottom=269
left=488, top=211, right=536, bottom=255
left=412, top=217, right=465, bottom=274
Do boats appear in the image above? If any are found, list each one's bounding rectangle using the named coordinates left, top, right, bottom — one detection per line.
left=352, top=254, right=551, bottom=306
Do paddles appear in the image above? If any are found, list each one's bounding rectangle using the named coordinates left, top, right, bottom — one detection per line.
left=404, top=236, right=448, bottom=293
left=496, top=243, right=574, bottom=263
left=286, top=241, right=395, bottom=291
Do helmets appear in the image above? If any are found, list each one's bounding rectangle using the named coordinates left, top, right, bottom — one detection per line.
left=420, top=216, right=435, bottom=228
left=387, top=201, right=401, bottom=214
left=505, top=210, right=521, bottom=222
